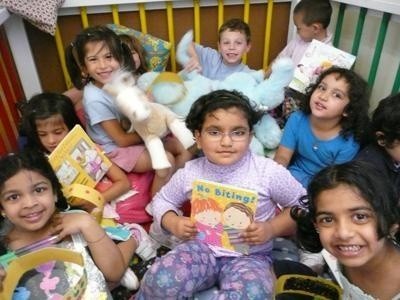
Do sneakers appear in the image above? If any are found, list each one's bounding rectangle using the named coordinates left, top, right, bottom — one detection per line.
left=128, top=222, right=157, bottom=263
left=120, top=267, right=140, bottom=292
left=298, top=242, right=324, bottom=276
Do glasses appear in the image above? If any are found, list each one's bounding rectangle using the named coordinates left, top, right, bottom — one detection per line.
left=207, top=131, right=253, bottom=142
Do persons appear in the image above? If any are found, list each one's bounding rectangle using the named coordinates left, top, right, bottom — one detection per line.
left=0, top=1, right=400, bottom=300
